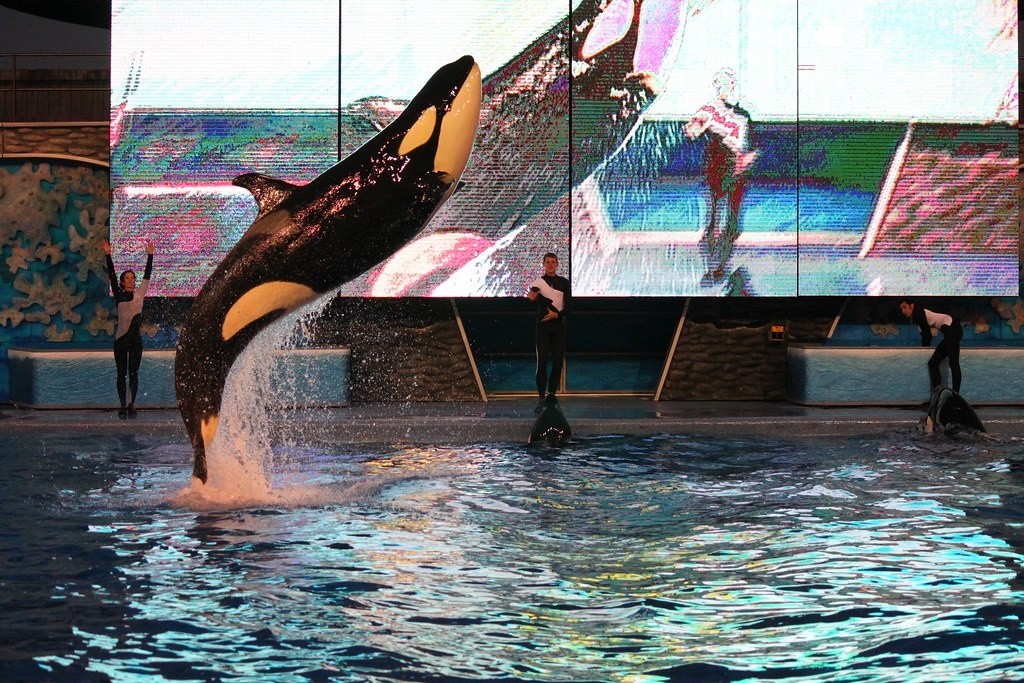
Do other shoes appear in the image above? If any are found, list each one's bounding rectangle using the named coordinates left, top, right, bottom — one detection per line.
left=534, top=398, right=546, bottom=416
left=918, top=399, right=932, bottom=412
left=117, top=406, right=127, bottom=420
left=127, top=403, right=138, bottom=419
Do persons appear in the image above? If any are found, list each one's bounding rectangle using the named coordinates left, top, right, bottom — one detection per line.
left=523, top=253, right=573, bottom=412
left=102, top=238, right=155, bottom=417
left=900, top=296, right=962, bottom=408
